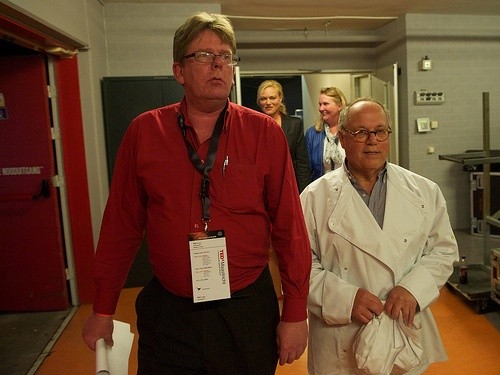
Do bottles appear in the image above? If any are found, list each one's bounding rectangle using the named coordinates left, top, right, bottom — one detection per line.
left=460, top=256, right=467, bottom=284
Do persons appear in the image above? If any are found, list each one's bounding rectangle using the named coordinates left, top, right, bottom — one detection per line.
left=296, top=96, right=459, bottom=375
left=83, top=10, right=312, bottom=375
left=254, top=78, right=311, bottom=322
left=306, top=86, right=348, bottom=186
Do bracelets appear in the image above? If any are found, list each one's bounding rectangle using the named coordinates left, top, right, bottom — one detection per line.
left=95, top=312, right=115, bottom=317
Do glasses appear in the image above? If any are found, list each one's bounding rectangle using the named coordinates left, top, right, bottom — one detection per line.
left=181, top=50, right=241, bottom=66
left=341, top=126, right=392, bottom=143
